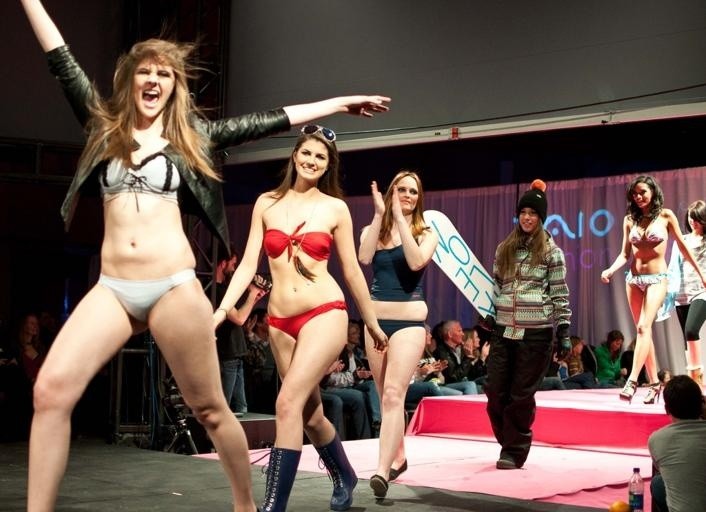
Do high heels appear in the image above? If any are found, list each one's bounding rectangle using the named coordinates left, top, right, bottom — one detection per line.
left=619, top=379, right=639, bottom=404
left=643, top=379, right=662, bottom=404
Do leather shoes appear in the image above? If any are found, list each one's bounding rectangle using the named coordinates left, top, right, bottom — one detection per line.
left=370, top=458, right=407, bottom=497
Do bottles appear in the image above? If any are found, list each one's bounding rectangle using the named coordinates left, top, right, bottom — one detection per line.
left=627, top=467, right=645, bottom=512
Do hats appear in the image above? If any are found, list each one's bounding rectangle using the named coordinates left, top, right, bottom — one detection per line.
left=516, top=178, right=547, bottom=225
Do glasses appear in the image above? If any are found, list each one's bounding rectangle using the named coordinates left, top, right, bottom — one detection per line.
left=300, top=122, right=337, bottom=143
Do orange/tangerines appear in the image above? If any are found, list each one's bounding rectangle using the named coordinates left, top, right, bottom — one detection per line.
left=607, top=501, right=630, bottom=512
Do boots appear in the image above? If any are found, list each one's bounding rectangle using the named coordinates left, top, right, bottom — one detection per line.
left=314, top=429, right=358, bottom=510
left=255, top=445, right=301, bottom=512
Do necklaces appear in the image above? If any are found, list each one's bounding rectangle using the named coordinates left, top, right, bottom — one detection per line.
left=286, top=191, right=317, bottom=283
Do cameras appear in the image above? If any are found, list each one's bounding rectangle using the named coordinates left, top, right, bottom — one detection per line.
left=251, top=273, right=272, bottom=291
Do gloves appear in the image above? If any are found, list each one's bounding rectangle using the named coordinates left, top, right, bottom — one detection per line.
left=556, top=324, right=572, bottom=362
left=477, top=314, right=499, bottom=333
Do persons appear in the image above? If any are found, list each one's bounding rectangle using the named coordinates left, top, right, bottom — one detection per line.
left=482, top=179, right=572, bottom=469
left=204, top=123, right=390, bottom=512
left=647, top=374, right=706, bottom=512
left=409, top=321, right=491, bottom=396
left=16, top=313, right=45, bottom=368
left=21, top=0, right=391, bottom=512
left=550, top=331, right=669, bottom=387
left=322, top=320, right=382, bottom=438
left=204, top=250, right=281, bottom=415
left=658, top=199, right=706, bottom=385
left=359, top=172, right=436, bottom=498
left=600, top=175, right=706, bottom=405
